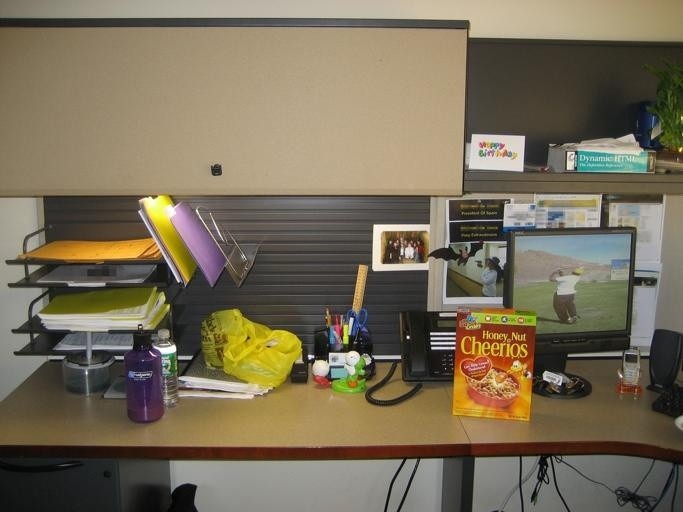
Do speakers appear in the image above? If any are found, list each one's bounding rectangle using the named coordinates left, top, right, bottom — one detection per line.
left=646, top=329, right=683, bottom=393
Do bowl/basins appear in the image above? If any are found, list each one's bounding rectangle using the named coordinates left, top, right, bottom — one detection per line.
left=465, top=368, right=522, bottom=409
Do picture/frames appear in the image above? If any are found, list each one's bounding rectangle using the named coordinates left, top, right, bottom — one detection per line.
left=371, top=223, right=430, bottom=274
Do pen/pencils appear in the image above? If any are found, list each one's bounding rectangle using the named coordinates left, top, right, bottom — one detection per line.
left=324, top=307, right=354, bottom=344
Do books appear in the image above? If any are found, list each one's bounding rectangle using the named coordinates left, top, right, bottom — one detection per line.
left=175, top=349, right=269, bottom=398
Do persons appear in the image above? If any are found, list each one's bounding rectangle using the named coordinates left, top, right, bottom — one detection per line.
left=385, top=238, right=423, bottom=264
left=549, top=268, right=584, bottom=324
left=481, top=256, right=502, bottom=296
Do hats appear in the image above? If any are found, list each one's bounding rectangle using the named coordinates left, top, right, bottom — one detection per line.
left=486, top=256, right=497, bottom=264
left=573, top=268, right=583, bottom=274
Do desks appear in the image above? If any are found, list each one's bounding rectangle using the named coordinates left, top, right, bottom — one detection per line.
left=0, top=352, right=683, bottom=511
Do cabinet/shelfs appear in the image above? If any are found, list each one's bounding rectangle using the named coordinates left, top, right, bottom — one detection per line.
left=3, top=219, right=190, bottom=361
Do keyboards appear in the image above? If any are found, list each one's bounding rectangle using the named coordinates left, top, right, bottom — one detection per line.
left=652, top=387, right=683, bottom=417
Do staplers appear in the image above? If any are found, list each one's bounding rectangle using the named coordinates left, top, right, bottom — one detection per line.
left=290, top=345, right=308, bottom=382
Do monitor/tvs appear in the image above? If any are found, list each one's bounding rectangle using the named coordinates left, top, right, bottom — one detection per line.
left=503, top=226, right=637, bottom=400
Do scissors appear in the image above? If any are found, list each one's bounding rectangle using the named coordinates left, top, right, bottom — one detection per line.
left=346, top=309, right=368, bottom=335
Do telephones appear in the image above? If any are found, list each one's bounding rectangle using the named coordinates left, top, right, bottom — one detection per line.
left=400, top=310, right=458, bottom=382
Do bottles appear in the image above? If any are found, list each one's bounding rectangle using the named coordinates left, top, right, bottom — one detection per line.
left=153, top=330, right=180, bottom=408
left=124, top=330, right=165, bottom=424
